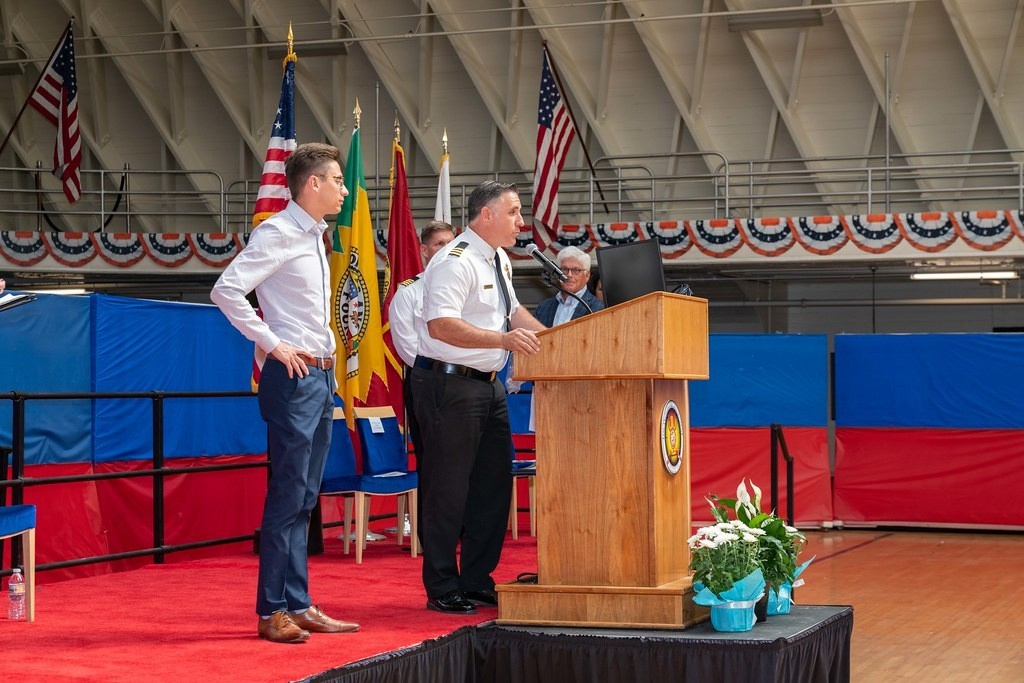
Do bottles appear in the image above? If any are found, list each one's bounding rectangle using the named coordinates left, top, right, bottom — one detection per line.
left=8, top=569, right=26, bottom=620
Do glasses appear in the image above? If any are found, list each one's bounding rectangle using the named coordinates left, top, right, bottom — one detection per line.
left=561, top=268, right=586, bottom=275
left=307, top=173, right=344, bottom=188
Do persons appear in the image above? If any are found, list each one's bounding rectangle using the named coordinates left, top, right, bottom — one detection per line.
left=210, top=142, right=362, bottom=642
left=532, top=245, right=604, bottom=328
left=388, top=180, right=548, bottom=614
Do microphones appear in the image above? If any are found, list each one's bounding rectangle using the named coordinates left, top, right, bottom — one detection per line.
left=526, top=244, right=568, bottom=283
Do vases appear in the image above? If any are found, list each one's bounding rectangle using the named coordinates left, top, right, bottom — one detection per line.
left=709, top=600, right=755, bottom=631
left=754, top=580, right=771, bottom=623
left=765, top=579, right=793, bottom=616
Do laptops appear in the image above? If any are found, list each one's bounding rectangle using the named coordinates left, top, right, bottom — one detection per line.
left=596, top=237, right=667, bottom=308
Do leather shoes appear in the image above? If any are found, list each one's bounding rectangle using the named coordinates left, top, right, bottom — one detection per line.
left=258, top=610, right=311, bottom=642
left=463, top=587, right=498, bottom=608
left=427, top=590, right=478, bottom=614
left=291, top=605, right=361, bottom=633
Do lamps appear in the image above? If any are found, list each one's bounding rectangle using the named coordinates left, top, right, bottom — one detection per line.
left=0, top=47, right=29, bottom=76
left=726, top=2, right=835, bottom=32
left=266, top=24, right=354, bottom=59
left=910, top=270, right=1021, bottom=280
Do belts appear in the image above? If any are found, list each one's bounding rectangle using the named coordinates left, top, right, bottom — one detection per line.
left=270, top=353, right=332, bottom=370
left=413, top=354, right=497, bottom=383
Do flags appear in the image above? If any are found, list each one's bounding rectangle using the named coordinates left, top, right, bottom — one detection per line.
left=27, top=20, right=83, bottom=204
left=329, top=122, right=451, bottom=436
left=532, top=48, right=577, bottom=254
left=251, top=52, right=297, bottom=394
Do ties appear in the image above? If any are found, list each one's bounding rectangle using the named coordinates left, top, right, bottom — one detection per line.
left=495, top=252, right=512, bottom=353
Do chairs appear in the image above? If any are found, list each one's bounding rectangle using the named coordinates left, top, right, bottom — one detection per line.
left=0, top=504, right=38, bottom=622
left=507, top=438, right=537, bottom=539
left=315, top=408, right=419, bottom=564
left=353, top=406, right=422, bottom=553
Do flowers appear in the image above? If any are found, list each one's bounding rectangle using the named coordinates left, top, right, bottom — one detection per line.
left=688, top=475, right=809, bottom=603
